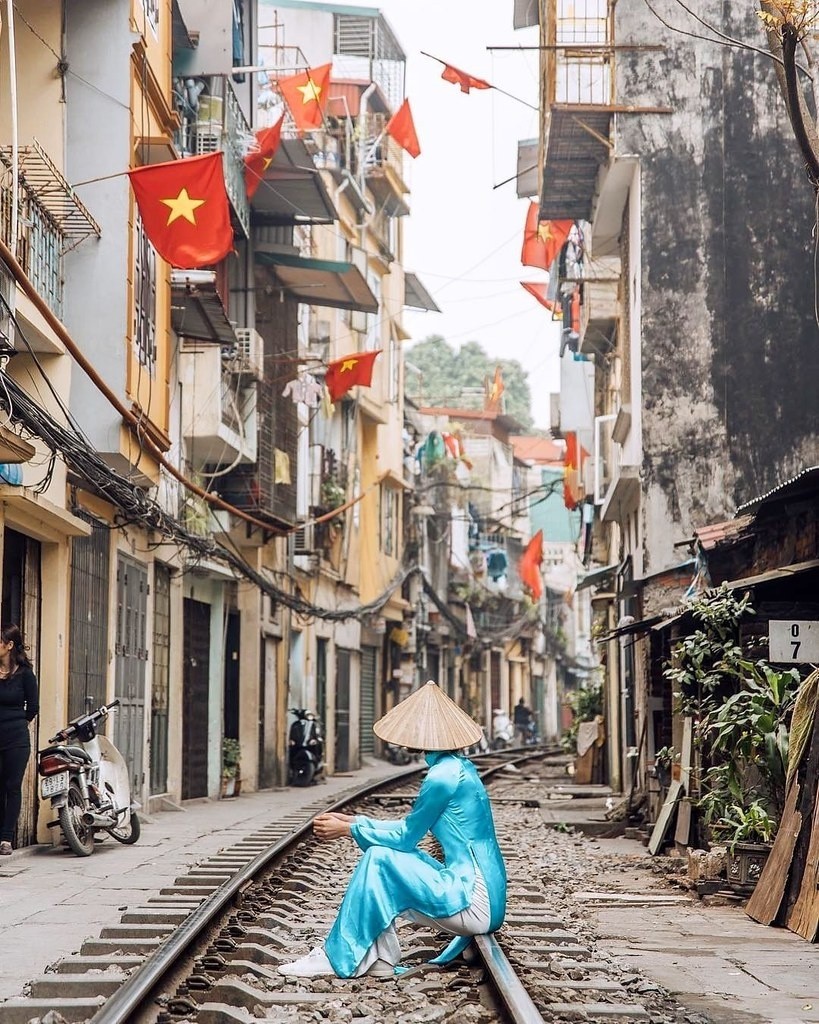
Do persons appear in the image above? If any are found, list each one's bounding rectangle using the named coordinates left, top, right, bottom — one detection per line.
left=276, top=680, right=507, bottom=978
left=0, top=619, right=39, bottom=856
left=513, top=697, right=540, bottom=746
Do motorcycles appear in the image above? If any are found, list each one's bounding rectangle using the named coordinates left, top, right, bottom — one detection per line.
left=32, top=696, right=143, bottom=858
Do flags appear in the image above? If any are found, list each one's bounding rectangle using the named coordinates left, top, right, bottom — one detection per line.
left=520, top=201, right=574, bottom=273
left=243, top=113, right=285, bottom=203
left=127, top=151, right=235, bottom=270
left=278, top=61, right=334, bottom=139
left=519, top=528, right=545, bottom=604
left=519, top=280, right=580, bottom=332
left=325, top=349, right=380, bottom=403
left=441, top=63, right=492, bottom=94
left=489, top=366, right=505, bottom=410
left=563, top=432, right=591, bottom=511
left=385, top=97, right=421, bottom=159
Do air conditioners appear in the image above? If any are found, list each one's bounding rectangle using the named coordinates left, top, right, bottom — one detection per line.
left=231, top=328, right=264, bottom=382
left=295, top=516, right=315, bottom=555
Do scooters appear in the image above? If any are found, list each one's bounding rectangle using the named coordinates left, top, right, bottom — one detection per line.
left=287, top=708, right=328, bottom=788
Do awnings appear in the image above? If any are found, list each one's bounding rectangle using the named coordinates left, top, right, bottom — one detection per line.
left=651, top=559, right=819, bottom=631
left=597, top=615, right=663, bottom=645
left=513, top=0, right=540, bottom=31
left=516, top=136, right=542, bottom=198
left=404, top=272, right=442, bottom=313
left=574, top=564, right=618, bottom=591
left=255, top=249, right=380, bottom=314
left=246, top=137, right=340, bottom=221
left=170, top=269, right=239, bottom=344
left=733, top=465, right=819, bottom=518
left=134, top=135, right=179, bottom=166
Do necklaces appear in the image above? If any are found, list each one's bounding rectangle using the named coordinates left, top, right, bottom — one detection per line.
left=0, top=668, right=10, bottom=674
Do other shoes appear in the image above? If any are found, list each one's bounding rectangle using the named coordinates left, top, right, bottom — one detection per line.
left=0, top=843, right=12, bottom=855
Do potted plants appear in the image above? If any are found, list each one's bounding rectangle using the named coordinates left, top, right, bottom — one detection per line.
left=652, top=581, right=801, bottom=898
left=220, top=737, right=241, bottom=798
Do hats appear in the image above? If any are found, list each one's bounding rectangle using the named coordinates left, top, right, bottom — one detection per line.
left=373, top=680, right=483, bottom=751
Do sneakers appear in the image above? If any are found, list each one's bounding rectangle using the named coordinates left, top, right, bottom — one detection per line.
left=276, top=947, right=336, bottom=976
left=365, top=960, right=395, bottom=977
